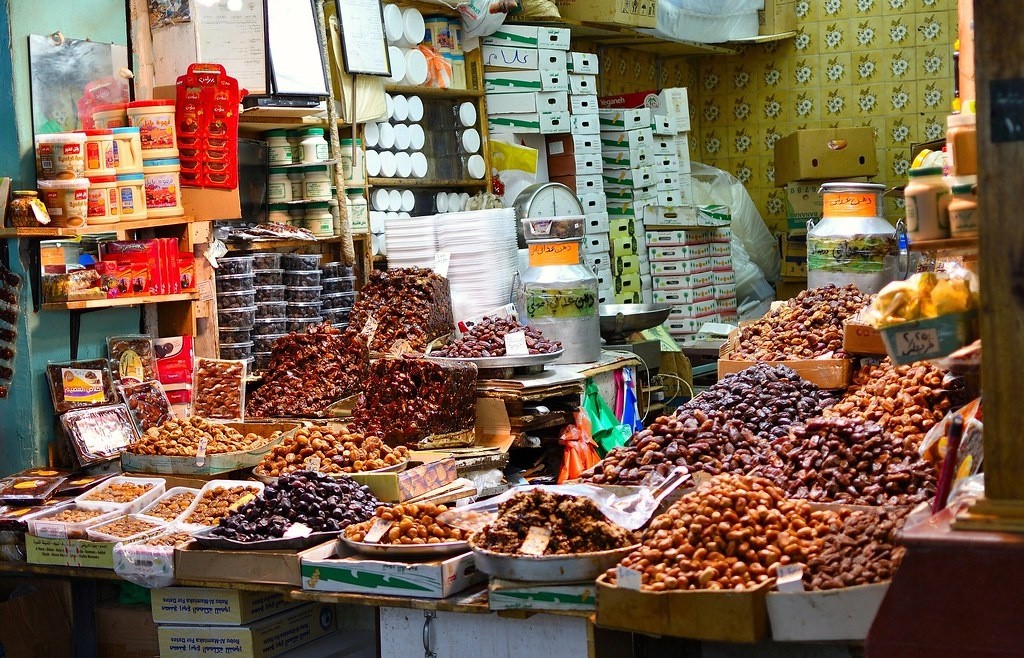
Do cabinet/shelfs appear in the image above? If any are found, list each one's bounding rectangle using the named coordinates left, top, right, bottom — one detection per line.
left=320, top=0, right=492, bottom=261
left=8, top=217, right=201, bottom=310
left=152, top=83, right=372, bottom=383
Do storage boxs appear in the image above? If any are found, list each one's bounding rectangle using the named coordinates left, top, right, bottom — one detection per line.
left=0, top=1, right=976, bottom=658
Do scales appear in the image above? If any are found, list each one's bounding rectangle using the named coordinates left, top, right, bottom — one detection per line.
left=512, top=182, right=675, bottom=372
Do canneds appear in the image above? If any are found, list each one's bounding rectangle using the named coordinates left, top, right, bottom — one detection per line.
left=8, top=189, right=39, bottom=227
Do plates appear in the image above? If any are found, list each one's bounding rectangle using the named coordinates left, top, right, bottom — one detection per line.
left=339, top=529, right=468, bottom=554
left=422, top=349, right=564, bottom=368
left=469, top=518, right=642, bottom=583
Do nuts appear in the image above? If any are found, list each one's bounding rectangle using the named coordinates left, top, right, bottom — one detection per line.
left=27, top=253, right=961, bottom=602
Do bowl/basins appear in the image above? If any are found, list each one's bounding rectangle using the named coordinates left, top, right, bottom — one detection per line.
left=600, top=303, right=674, bottom=340
